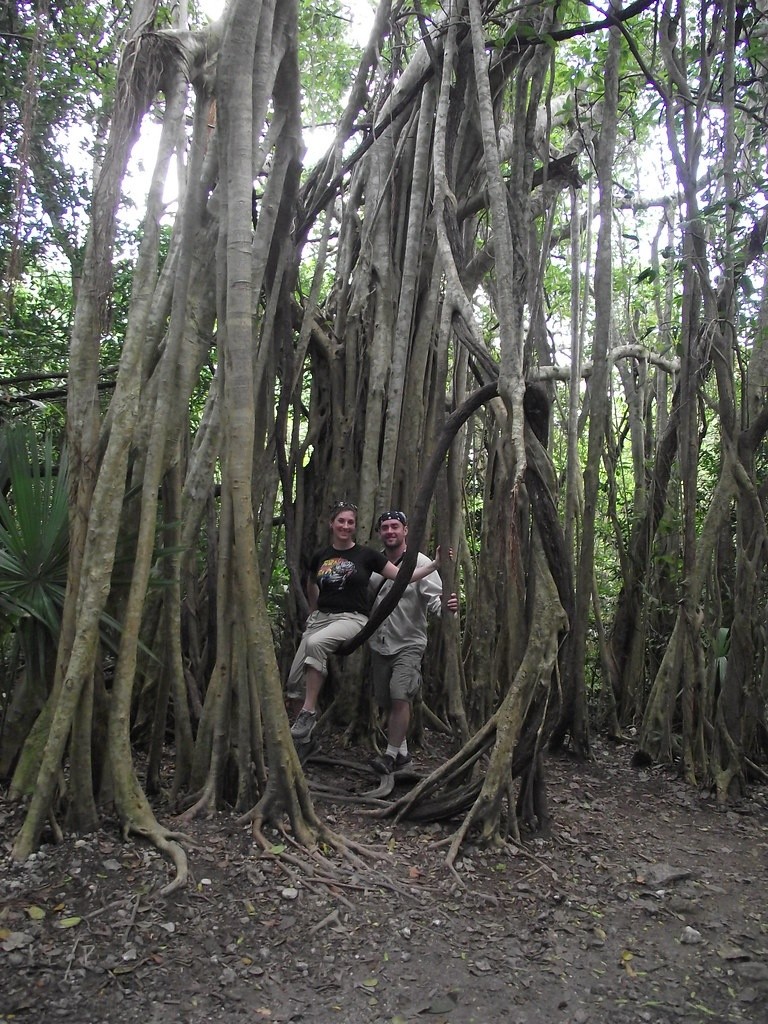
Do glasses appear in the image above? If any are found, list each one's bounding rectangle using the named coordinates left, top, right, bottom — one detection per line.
left=329, top=501, right=359, bottom=514
left=379, top=510, right=405, bottom=525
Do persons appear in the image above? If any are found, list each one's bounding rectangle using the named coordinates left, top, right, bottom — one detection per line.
left=364, top=512, right=458, bottom=774
left=285, top=502, right=454, bottom=745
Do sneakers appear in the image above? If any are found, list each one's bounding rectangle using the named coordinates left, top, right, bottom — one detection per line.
left=369, top=751, right=411, bottom=773
left=289, top=707, right=316, bottom=744
